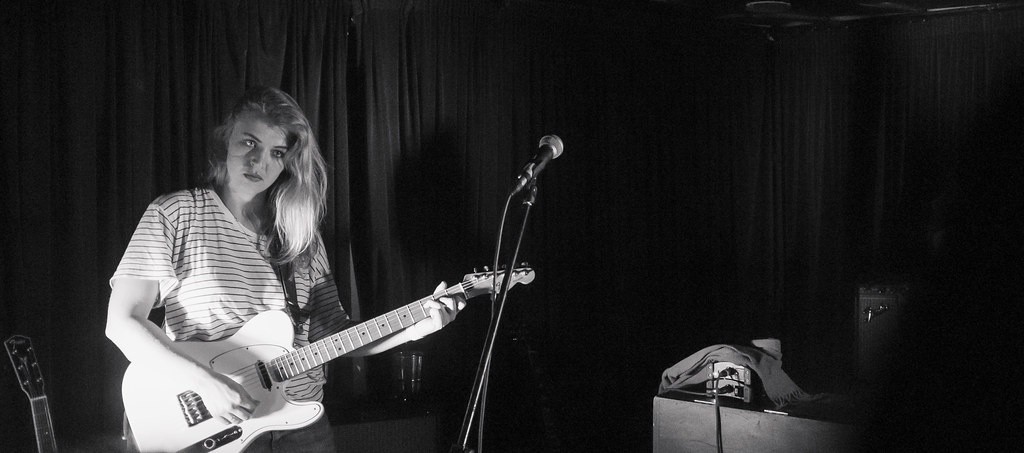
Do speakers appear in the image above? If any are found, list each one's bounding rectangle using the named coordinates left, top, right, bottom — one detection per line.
left=856, top=279, right=920, bottom=381
left=323, top=394, right=445, bottom=453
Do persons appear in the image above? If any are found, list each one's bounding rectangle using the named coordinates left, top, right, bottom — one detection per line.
left=105, top=83, right=467, bottom=453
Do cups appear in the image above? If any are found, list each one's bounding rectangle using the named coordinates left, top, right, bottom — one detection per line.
left=391, top=350, right=425, bottom=419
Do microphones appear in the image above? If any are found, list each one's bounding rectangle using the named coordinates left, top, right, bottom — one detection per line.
left=509, top=135, right=564, bottom=196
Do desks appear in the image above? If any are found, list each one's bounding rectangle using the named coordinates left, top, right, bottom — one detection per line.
left=332, top=401, right=439, bottom=453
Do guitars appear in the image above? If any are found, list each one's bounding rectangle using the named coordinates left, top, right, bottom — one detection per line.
left=3, top=334, right=58, bottom=453
left=121, top=261, right=536, bottom=453
